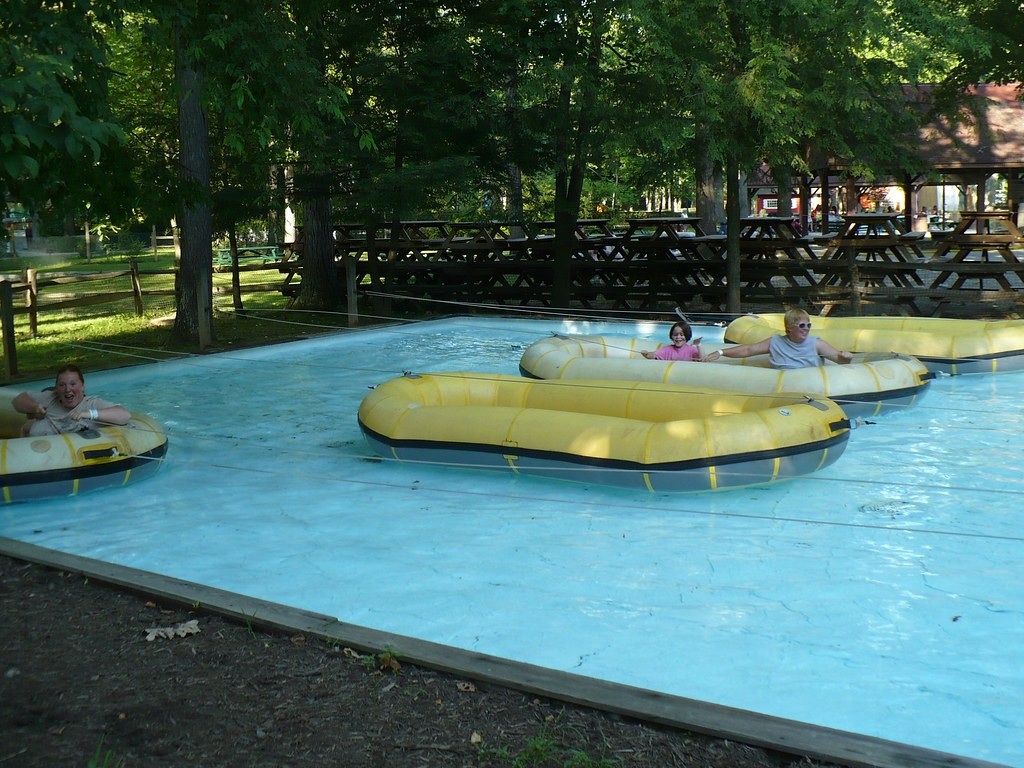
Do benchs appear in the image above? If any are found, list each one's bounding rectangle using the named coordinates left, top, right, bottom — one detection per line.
left=262, top=232, right=1024, bottom=319
left=213, top=255, right=283, bottom=266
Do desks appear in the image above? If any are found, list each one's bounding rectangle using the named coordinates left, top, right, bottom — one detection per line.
left=294, top=211, right=1024, bottom=291
left=218, top=246, right=279, bottom=266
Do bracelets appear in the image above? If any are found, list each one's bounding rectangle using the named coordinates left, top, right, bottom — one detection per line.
left=697, top=343, right=701, bottom=346
left=89, top=409, right=98, bottom=421
left=717, top=349, right=723, bottom=355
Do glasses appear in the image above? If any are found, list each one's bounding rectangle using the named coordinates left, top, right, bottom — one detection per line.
left=793, top=324, right=812, bottom=329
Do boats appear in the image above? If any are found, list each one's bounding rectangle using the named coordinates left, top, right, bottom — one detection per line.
left=356, top=369, right=853, bottom=493
left=518, top=331, right=937, bottom=420
left=722, top=311, right=1024, bottom=376
left=1, top=389, right=170, bottom=510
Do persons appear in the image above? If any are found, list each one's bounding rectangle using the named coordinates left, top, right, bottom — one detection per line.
left=917, top=206, right=938, bottom=217
left=681, top=210, right=690, bottom=230
left=703, top=308, right=853, bottom=369
left=791, top=216, right=802, bottom=234
left=641, top=322, right=704, bottom=361
left=887, top=206, right=894, bottom=213
left=812, top=205, right=838, bottom=223
left=12, top=365, right=131, bottom=436
